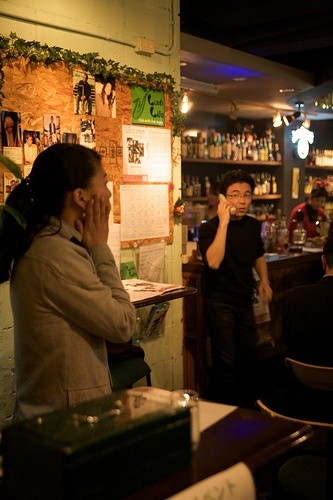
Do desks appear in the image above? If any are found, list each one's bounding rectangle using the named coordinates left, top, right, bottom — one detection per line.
left=0, top=399, right=303, bottom=500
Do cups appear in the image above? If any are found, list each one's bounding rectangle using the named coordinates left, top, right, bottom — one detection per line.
left=169, top=389, right=200, bottom=450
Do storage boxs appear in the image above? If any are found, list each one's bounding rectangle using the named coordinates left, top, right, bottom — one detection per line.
left=19, top=387, right=193, bottom=499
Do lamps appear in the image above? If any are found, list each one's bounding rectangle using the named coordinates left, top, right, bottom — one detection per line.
left=284, top=101, right=303, bottom=126
left=228, top=101, right=239, bottom=120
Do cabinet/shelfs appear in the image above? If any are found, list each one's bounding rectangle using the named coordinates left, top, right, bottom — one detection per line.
left=182, top=156, right=281, bottom=201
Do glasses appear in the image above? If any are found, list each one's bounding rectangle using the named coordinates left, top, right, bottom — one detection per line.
left=227, top=194, right=252, bottom=199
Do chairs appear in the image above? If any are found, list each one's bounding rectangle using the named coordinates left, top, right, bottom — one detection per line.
left=255, top=357, right=332, bottom=429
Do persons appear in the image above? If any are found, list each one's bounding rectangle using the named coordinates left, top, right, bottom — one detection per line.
left=198, top=170, right=273, bottom=409
left=280, top=236, right=333, bottom=368
left=24, top=134, right=37, bottom=164
left=49, top=116, right=56, bottom=134
left=288, top=187, right=329, bottom=240
left=3, top=117, right=16, bottom=146
left=75, top=73, right=92, bottom=114
left=0, top=142, right=136, bottom=425
left=96, top=82, right=113, bottom=117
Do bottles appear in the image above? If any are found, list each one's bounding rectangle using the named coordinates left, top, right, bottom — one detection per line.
left=306, top=147, right=333, bottom=197
left=183, top=170, right=278, bottom=198
left=182, top=200, right=331, bottom=253
left=182, top=130, right=282, bottom=163
